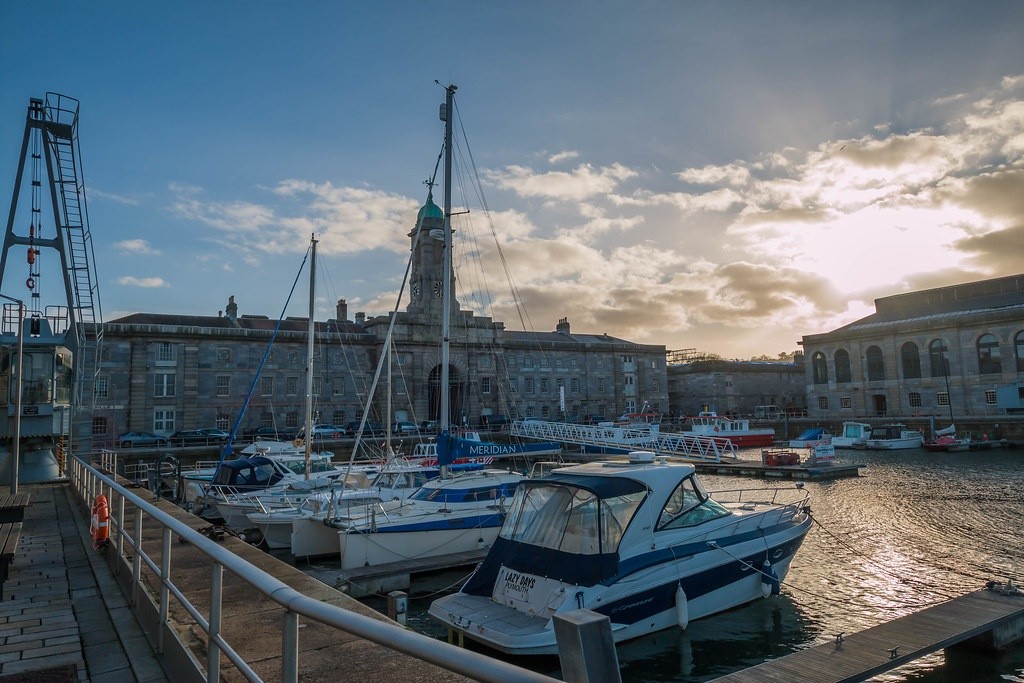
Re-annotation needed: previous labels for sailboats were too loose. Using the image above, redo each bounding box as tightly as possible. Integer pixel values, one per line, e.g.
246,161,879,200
205,84,642,573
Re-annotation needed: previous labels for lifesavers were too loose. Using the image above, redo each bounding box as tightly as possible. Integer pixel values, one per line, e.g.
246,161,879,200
89,494,109,551
292,438,304,449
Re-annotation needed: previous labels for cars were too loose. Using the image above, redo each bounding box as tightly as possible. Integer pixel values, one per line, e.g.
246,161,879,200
311,423,346,437
242,427,295,441
614,413,630,421
117,431,167,447
170,429,223,444
195,427,236,441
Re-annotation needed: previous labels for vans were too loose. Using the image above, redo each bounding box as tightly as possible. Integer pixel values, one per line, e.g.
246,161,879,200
420,419,441,433
585,414,606,425
393,421,421,433
754,406,784,419
346,422,387,438
480,415,511,431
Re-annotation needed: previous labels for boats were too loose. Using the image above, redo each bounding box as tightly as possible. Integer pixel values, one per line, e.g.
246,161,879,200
613,400,776,448
924,340,1007,451
162,231,514,552
427,452,816,658
831,421,872,450
867,423,923,448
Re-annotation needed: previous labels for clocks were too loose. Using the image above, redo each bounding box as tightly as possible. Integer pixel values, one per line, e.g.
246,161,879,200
431,281,444,299
411,282,420,299
